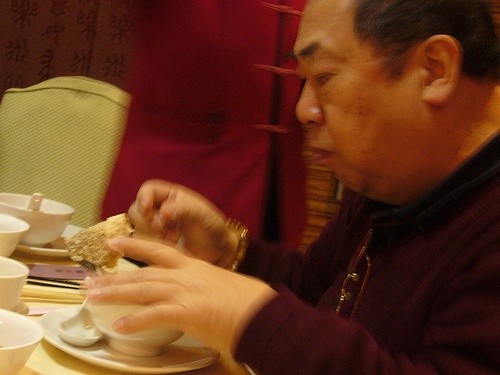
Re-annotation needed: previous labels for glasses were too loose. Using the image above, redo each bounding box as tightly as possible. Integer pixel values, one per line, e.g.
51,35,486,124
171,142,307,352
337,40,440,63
333,230,372,318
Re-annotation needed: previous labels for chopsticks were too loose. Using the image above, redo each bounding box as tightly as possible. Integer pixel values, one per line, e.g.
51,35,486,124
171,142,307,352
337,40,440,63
19,292,85,305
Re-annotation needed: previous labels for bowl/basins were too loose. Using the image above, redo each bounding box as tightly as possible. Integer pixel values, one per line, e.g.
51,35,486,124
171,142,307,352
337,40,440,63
84,299,187,358
0,214,30,258
0,307,45,375
0,256,31,310
0,193,75,247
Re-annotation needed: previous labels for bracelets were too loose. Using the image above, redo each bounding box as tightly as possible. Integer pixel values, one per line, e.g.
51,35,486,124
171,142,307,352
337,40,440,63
225,217,249,273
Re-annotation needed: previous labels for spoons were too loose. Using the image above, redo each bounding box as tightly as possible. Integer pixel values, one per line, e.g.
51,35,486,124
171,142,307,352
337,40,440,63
59,285,106,346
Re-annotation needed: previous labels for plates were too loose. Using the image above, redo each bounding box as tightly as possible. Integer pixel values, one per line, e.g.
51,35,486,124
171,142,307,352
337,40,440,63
40,306,221,373
17,225,90,256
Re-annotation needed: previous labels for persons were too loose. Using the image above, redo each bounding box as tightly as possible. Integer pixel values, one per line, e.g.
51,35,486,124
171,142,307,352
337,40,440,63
84,0,500,375
